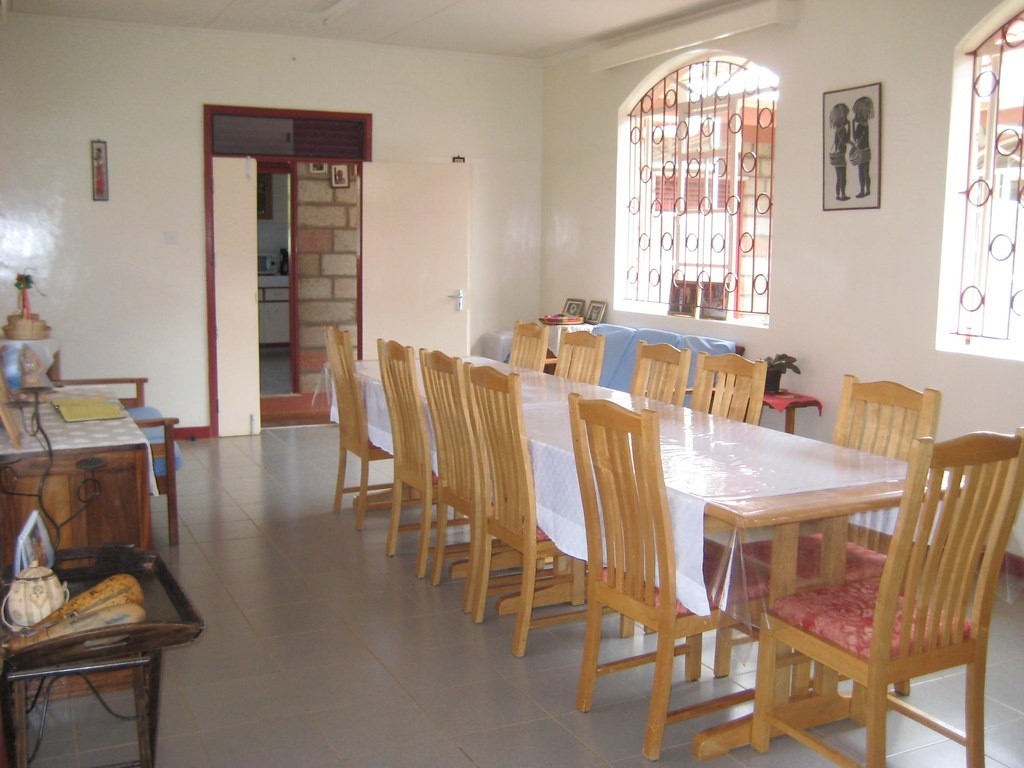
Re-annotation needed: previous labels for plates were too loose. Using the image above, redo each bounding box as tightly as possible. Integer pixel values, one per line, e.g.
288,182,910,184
538,318,584,325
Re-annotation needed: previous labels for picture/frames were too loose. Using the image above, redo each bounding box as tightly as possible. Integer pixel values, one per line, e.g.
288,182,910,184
330,162,352,189
668,279,730,321
583,300,607,324
822,82,881,212
562,298,586,316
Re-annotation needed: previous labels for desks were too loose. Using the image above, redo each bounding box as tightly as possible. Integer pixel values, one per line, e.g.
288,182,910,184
5,646,162,768
330,356,967,761
678,386,823,435
541,324,596,376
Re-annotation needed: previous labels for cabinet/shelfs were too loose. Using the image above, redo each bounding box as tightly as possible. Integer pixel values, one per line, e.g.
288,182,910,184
259,275,290,348
0,385,159,705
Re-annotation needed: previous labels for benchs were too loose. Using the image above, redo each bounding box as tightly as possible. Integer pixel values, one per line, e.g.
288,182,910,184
583,324,745,408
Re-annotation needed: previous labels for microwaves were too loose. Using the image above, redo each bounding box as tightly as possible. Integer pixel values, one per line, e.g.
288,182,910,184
258,253,277,274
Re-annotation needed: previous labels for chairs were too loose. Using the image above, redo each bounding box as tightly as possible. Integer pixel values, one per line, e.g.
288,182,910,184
325,319,1024,768
44,351,184,547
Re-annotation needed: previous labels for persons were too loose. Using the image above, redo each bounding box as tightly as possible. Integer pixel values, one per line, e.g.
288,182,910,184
570,304,576,313
336,170,346,184
674,286,693,312
589,306,597,320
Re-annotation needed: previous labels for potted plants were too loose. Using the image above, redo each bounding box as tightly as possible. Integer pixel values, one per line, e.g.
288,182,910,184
754,353,801,393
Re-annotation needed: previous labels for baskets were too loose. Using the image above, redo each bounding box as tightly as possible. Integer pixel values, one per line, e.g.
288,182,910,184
4,275,52,340
